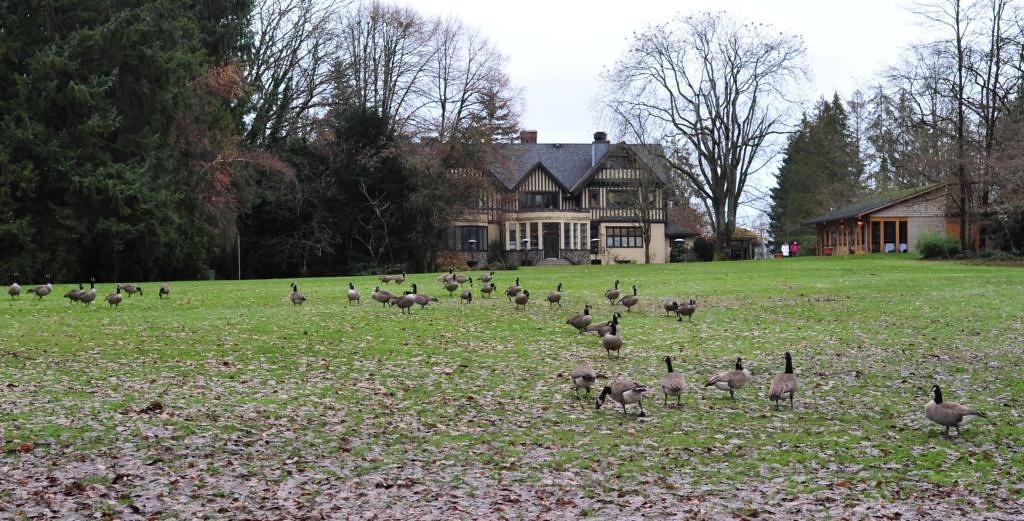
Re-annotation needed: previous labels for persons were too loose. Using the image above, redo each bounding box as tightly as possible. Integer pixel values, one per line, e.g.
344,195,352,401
781,241,790,258
791,241,799,256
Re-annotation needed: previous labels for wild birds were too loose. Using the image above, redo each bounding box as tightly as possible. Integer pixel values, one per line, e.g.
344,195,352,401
6,276,171,310
372,263,798,417
347,281,360,306
289,283,306,306
925,384,986,435
